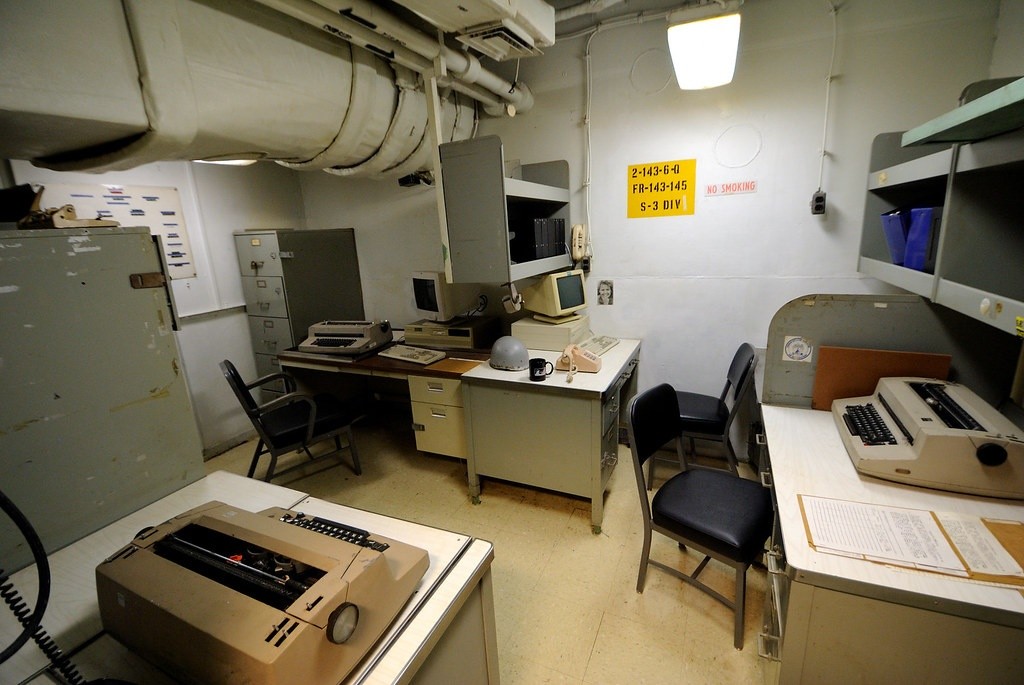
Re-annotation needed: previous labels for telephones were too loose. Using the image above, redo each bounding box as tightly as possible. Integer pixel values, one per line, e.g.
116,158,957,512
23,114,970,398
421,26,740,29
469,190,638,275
555,345,602,373
571,223,585,260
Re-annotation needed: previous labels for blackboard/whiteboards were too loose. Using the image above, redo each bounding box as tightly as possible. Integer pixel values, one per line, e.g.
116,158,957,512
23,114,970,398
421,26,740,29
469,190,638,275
41,181,197,280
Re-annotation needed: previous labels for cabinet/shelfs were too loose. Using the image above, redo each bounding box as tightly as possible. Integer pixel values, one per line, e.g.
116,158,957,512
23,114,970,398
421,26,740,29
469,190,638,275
437,135,574,288
857,115,1024,337
0,226,208,579
231,223,361,394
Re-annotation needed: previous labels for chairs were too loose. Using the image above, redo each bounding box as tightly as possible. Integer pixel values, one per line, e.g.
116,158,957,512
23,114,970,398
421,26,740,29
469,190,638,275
220,360,368,483
627,381,778,648
647,343,758,490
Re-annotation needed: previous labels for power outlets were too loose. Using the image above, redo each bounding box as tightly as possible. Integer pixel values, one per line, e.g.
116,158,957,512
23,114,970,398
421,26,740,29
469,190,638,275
812,191,826,215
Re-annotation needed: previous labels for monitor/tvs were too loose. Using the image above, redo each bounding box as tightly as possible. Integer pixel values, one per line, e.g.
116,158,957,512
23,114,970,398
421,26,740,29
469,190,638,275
411,271,485,327
522,269,588,325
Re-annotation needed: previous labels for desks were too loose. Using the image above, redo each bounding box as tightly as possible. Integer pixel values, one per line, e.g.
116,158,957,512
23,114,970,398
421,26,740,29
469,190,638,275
285,344,491,458
464,337,639,537
760,393,1024,685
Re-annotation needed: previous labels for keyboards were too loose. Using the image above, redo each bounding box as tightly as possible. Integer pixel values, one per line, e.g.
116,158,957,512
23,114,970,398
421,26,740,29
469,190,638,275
378,344,446,364
577,334,619,357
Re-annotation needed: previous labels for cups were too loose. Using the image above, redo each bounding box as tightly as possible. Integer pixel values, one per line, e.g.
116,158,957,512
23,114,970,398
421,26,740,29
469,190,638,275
529,358,554,382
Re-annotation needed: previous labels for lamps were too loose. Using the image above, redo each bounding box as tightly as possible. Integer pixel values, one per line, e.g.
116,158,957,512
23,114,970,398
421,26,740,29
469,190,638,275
665,3,743,91
